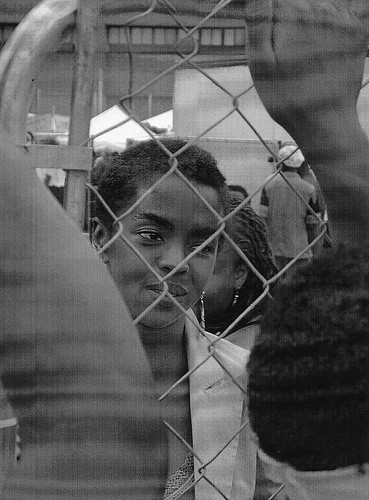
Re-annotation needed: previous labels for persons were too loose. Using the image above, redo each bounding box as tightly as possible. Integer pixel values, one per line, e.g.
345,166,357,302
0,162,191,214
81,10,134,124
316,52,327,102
0,0,369,500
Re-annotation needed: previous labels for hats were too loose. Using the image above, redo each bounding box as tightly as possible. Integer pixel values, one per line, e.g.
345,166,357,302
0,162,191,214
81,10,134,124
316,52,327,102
278,145,305,169
